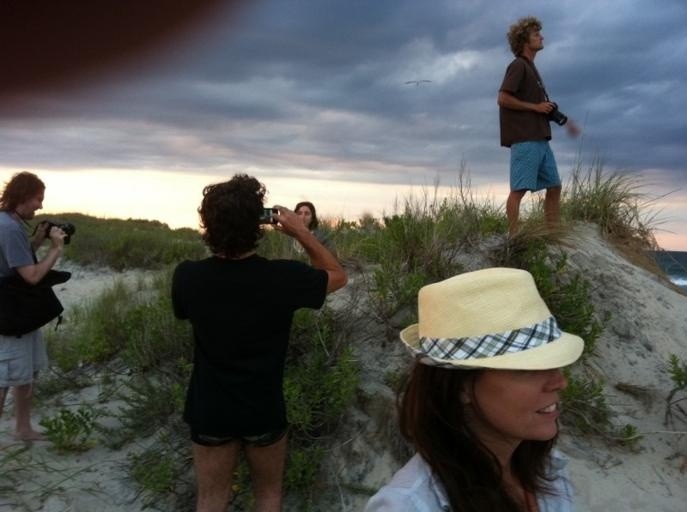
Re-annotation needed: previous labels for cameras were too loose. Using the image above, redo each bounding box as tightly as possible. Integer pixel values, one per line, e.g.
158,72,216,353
545,101,568,126
44,223,77,245
259,207,279,224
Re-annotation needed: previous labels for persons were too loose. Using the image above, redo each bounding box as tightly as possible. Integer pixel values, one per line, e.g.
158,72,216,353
170,175,347,511
362,268,585,512
495,15,563,240
291,203,337,269
0,171,68,442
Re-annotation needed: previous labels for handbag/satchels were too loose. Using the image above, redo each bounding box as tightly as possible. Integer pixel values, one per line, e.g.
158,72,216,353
0,269,71,338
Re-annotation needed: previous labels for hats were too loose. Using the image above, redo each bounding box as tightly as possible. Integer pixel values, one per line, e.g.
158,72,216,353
399,267,584,371
